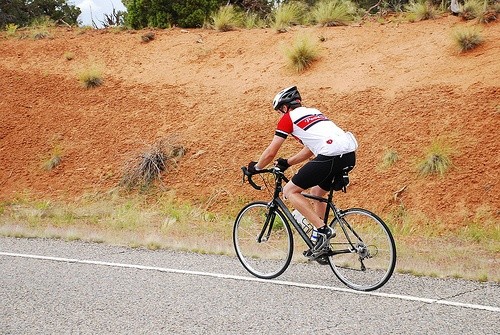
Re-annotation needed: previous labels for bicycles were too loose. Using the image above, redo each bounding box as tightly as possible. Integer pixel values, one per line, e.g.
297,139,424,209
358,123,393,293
233,162,397,293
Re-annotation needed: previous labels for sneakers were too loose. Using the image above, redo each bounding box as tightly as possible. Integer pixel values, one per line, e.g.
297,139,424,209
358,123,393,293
303,249,328,265
314,225,336,250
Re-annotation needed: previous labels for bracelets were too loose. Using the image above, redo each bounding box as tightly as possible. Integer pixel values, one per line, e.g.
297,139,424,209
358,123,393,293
254,164,263,171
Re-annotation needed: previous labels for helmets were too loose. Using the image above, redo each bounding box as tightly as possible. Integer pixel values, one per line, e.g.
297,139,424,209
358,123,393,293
272,86,301,111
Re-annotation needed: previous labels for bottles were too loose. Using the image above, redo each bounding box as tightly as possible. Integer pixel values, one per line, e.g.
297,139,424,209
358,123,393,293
292,209,313,233
310,217,324,241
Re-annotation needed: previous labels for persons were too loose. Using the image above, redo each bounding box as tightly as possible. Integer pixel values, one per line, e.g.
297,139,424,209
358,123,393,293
247,85,358,257
